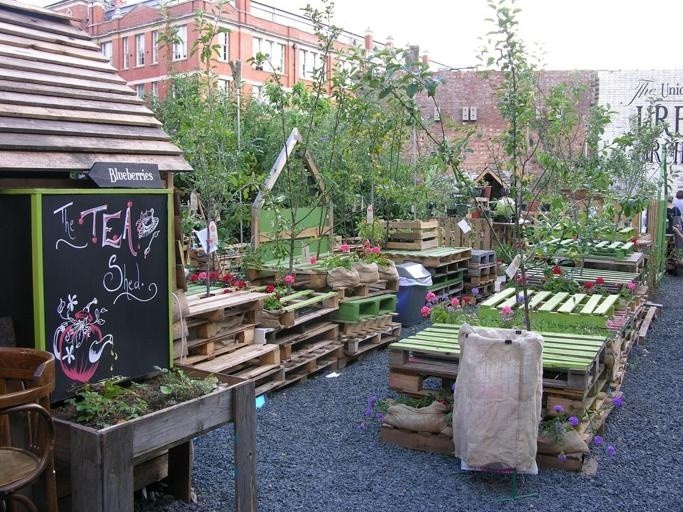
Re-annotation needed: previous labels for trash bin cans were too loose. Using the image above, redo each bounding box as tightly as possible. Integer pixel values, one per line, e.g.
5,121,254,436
392,262,432,328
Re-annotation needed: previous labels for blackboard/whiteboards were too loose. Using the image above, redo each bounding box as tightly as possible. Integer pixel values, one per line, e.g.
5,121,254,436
30,188,175,405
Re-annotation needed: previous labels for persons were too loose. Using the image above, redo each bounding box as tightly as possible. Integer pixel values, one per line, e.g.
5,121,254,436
495,187,517,223
672,189,683,217
666,195,681,254
